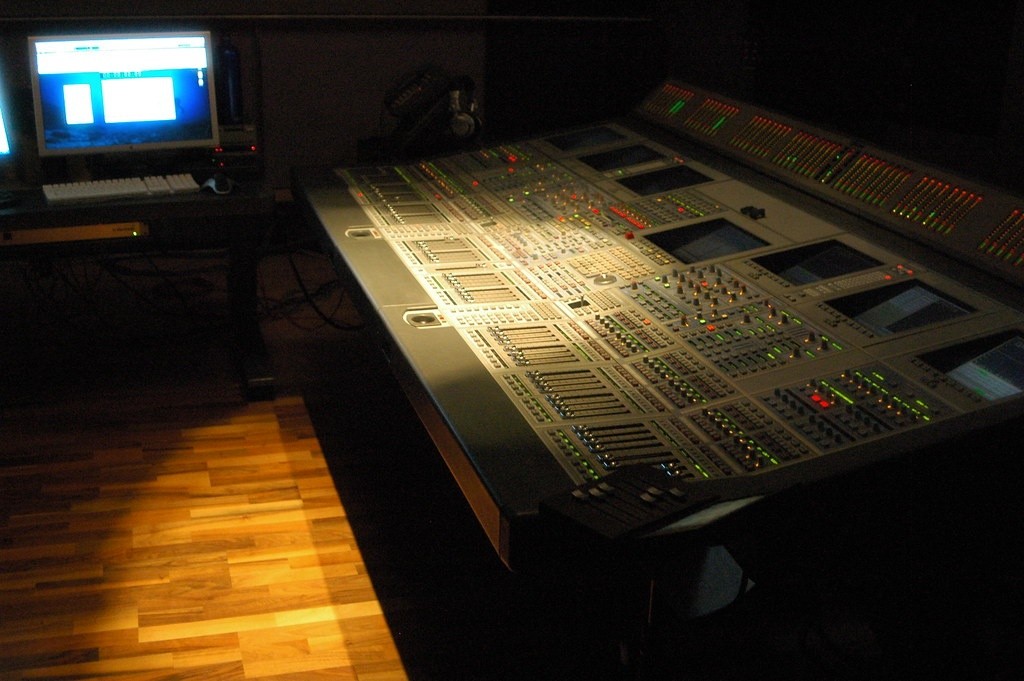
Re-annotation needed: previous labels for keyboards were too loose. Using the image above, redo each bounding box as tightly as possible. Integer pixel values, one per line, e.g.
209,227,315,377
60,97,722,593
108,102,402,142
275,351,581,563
42,173,200,207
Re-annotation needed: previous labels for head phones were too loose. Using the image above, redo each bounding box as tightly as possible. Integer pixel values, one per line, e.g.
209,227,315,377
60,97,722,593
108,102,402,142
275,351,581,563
446,74,485,138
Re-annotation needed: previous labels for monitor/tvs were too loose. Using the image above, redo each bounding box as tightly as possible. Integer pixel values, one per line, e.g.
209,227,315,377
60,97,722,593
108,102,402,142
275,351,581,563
28,29,220,157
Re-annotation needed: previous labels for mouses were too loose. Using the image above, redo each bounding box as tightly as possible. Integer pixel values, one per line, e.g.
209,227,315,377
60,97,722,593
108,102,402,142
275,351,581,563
201,176,233,194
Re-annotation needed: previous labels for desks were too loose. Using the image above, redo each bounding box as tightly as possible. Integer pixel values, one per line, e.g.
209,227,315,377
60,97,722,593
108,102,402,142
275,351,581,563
0,183,278,403
